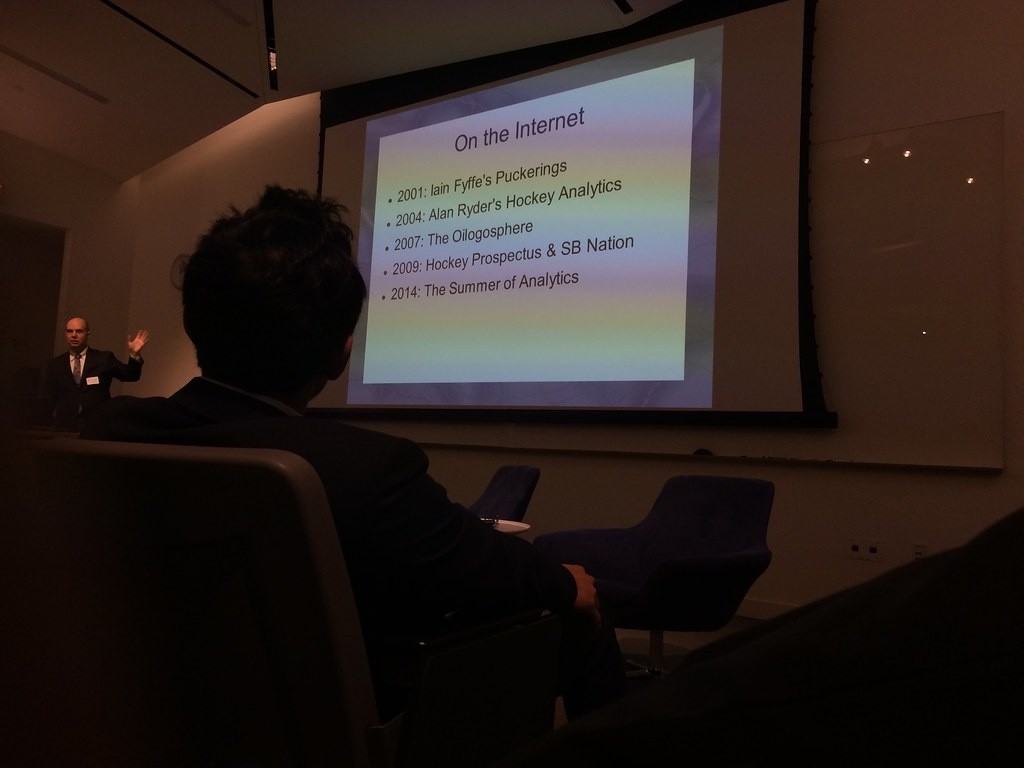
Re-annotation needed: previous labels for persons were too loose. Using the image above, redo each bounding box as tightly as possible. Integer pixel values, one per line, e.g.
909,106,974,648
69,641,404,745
39,317,152,400
78,186,622,725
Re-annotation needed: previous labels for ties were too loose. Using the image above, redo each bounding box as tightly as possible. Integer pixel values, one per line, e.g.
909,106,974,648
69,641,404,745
73,354,81,388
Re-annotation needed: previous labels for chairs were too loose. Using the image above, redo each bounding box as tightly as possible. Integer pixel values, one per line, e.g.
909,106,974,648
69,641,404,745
533,476,775,676
470,464,543,519
1,433,405,767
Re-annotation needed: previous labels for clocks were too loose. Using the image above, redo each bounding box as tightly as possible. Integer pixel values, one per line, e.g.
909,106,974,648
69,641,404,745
171,252,190,290
